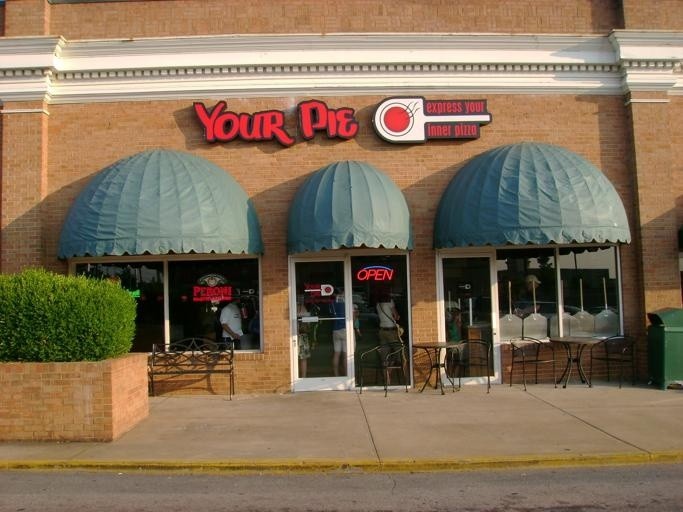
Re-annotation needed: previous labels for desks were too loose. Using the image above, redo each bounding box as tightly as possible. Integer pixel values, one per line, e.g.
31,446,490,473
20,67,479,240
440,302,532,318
412,341,464,396
549,336,601,388
462,321,493,377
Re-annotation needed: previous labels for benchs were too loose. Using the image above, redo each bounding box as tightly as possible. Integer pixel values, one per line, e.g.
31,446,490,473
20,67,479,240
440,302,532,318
146,338,235,402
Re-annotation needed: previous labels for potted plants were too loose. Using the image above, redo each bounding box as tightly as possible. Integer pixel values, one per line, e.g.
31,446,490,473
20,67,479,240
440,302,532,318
0,267,150,442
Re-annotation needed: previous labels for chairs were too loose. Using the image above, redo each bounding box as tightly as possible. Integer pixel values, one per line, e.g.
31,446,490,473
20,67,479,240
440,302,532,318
588,334,638,390
509,336,559,390
360,342,408,397
451,338,492,394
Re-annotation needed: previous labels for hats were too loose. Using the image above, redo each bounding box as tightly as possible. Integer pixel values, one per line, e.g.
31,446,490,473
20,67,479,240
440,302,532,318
524,274,541,284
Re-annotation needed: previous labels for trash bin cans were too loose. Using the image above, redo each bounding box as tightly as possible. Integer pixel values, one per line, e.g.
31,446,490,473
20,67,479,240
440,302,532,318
647,308,683,391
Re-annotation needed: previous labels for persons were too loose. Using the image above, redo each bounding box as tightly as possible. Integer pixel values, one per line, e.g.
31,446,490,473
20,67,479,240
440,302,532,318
446,306,468,378
218,291,245,352
352,302,362,338
328,288,346,377
295,293,312,378
512,274,541,318
375,285,410,386
